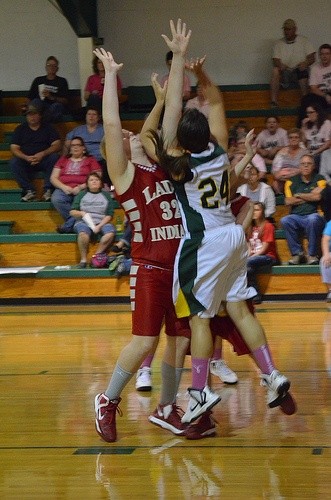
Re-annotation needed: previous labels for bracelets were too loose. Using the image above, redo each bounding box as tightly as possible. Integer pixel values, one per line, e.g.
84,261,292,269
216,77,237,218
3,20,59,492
320,93,326,97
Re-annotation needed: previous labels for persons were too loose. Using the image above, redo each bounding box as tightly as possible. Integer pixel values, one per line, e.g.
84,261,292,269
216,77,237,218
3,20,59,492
160,50,192,101
269,17,316,107
48,136,102,232
236,167,276,224
245,201,280,303
139,52,291,424
64,109,114,190
185,79,211,118
93,18,193,440
232,133,268,182
28,56,71,123
9,106,62,201
114,221,133,253
233,105,331,302
228,120,256,159
135,128,298,439
70,172,115,267
82,54,125,112
297,44,331,128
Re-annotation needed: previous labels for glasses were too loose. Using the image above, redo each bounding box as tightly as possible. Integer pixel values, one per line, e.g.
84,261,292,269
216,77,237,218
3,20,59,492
46,64,57,67
306,111,315,114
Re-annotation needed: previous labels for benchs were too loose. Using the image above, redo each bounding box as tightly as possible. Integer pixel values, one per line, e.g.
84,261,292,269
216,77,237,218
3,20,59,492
0,80,329,299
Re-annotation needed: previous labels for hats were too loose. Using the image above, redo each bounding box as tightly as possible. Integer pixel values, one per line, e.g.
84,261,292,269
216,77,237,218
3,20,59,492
280,18,297,30
24,105,41,114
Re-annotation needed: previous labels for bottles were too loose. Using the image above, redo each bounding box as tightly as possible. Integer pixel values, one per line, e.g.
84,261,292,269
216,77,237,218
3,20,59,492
115,216,122,233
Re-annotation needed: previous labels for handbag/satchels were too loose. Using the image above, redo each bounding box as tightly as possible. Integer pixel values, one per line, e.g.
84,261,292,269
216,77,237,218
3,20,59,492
91,254,107,267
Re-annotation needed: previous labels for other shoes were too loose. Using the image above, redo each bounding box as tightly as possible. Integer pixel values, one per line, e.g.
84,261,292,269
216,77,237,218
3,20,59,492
327,293,331,301
270,101,277,108
252,293,263,304
78,262,86,269
108,255,125,271
307,256,318,265
288,255,303,264
58,227,72,234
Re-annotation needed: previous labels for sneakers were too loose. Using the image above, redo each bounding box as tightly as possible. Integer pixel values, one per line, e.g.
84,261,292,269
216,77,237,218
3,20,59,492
136,366,152,391
21,191,38,201
259,369,290,408
209,360,239,384
185,412,216,439
149,402,185,435
94,392,122,442
42,191,51,201
280,394,296,415
180,385,222,424
